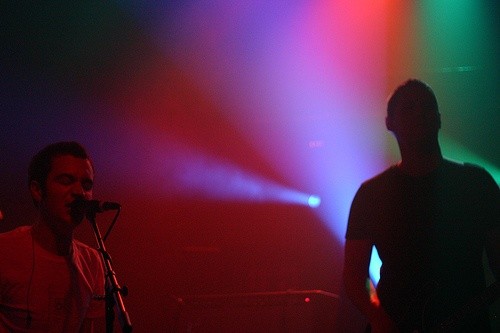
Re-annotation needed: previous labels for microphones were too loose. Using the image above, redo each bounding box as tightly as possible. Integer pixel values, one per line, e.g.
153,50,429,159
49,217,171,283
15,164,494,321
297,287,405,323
71,199,121,212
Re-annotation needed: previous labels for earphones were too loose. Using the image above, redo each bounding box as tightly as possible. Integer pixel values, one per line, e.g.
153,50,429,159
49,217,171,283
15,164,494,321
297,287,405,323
24,315,32,320
